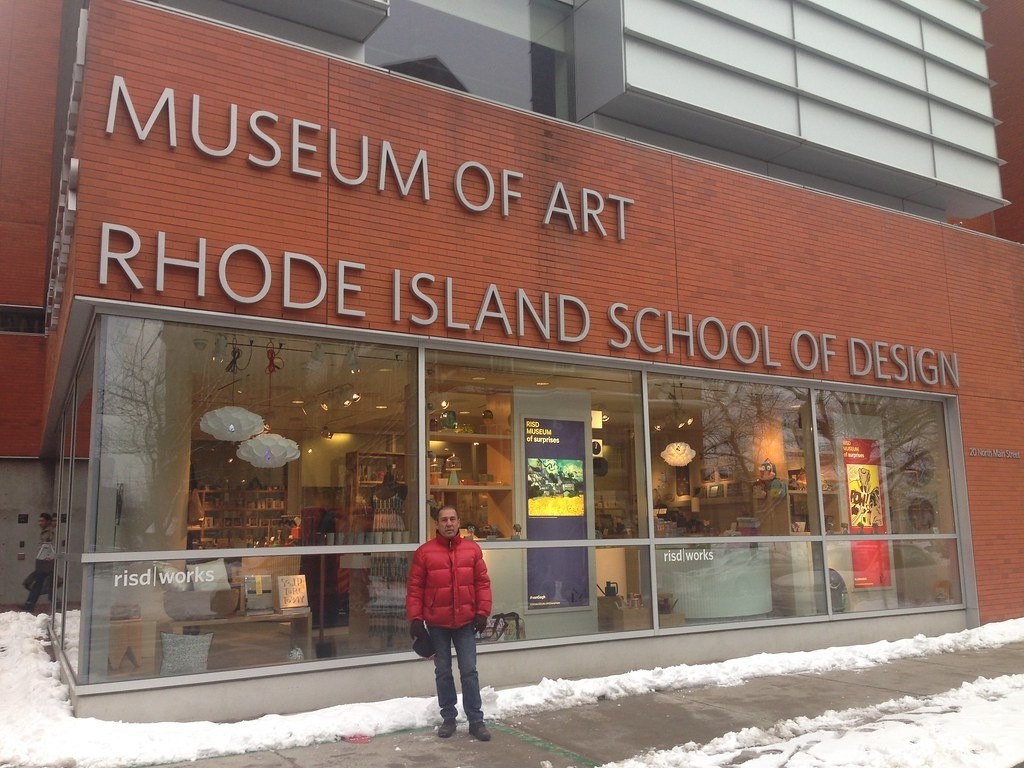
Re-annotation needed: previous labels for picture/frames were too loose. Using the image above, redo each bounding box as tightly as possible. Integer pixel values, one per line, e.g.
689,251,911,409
233,516,242,527
266,497,274,509
692,485,707,499
204,517,213,527
676,464,690,498
224,517,233,528
249,515,259,527
717,464,733,482
700,467,715,483
727,483,739,497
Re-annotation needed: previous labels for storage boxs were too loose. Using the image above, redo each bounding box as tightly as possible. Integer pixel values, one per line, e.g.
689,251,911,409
276,575,309,609
107,618,144,676
598,595,624,621
613,606,651,635
657,612,686,629
479,474,493,482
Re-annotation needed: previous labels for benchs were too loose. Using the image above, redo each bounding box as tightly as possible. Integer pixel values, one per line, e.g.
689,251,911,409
158,610,312,675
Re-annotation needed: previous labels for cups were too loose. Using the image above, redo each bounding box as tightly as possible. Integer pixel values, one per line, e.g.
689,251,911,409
393,531,402,543
438,478,448,485
374,531,383,543
402,531,410,543
335,533,344,544
383,532,392,543
366,532,374,544
326,533,334,545
796,522,806,532
356,532,364,544
345,532,354,544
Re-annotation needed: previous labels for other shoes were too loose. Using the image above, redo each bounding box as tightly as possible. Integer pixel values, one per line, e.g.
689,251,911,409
19,606,33,611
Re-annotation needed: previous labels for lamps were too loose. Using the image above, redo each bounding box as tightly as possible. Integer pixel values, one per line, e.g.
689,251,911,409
660,383,697,468
196,334,265,443
237,337,301,470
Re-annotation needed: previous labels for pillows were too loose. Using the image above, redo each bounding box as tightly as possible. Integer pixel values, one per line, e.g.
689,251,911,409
160,631,213,676
152,560,193,592
187,557,231,591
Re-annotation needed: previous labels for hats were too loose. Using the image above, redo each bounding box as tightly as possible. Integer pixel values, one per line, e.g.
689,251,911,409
410,628,435,657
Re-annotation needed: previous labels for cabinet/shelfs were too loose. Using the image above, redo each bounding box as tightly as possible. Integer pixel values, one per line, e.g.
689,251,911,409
188,489,287,544
405,377,513,540
765,408,899,615
345,451,458,537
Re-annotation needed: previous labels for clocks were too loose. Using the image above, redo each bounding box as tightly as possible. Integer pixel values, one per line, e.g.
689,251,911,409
592,438,603,458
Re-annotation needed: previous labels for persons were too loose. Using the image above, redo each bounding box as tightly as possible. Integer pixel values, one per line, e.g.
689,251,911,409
405,505,492,741
19,512,58,611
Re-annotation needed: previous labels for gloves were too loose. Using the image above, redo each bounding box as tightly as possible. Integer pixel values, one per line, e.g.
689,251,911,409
472,614,488,634
410,619,424,641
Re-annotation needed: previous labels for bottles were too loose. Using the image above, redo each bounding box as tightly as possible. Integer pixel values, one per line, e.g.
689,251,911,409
691,496,700,512
656,518,665,538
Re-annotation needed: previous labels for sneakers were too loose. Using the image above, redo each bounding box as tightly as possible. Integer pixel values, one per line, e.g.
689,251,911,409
438,718,457,737
469,721,491,742
